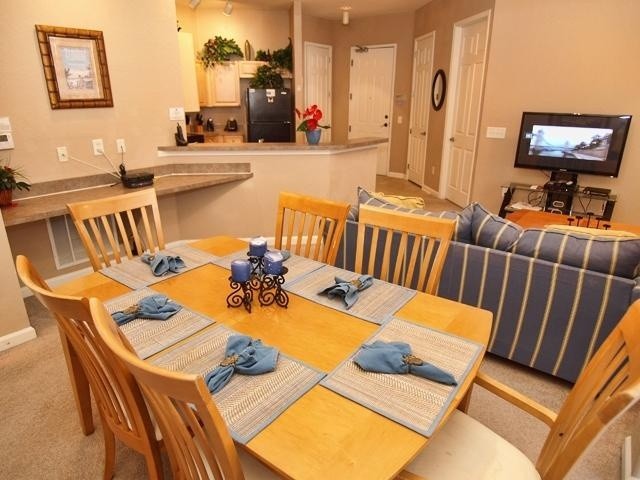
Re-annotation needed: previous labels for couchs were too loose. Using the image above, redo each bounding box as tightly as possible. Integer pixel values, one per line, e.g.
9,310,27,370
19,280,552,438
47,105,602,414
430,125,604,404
321,205,640,383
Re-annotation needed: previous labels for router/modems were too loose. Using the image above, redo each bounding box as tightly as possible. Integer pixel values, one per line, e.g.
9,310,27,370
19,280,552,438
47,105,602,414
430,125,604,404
583,186,612,198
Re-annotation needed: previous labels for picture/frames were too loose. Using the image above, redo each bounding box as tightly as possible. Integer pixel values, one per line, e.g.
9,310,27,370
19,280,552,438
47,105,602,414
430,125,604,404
35,24,115,110
432,69,447,111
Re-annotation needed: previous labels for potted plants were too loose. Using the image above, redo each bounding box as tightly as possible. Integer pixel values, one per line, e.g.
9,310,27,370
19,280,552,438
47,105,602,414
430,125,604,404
0,156,31,207
198,36,243,69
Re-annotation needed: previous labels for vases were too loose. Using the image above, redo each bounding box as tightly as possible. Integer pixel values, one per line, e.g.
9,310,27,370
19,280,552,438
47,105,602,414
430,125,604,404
306,129,322,144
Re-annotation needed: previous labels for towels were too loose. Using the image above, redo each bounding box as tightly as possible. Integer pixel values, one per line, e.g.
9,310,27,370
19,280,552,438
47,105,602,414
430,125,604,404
317,275,373,311
204,335,279,395
353,340,458,387
281,249,290,260
111,295,183,326
141,252,186,277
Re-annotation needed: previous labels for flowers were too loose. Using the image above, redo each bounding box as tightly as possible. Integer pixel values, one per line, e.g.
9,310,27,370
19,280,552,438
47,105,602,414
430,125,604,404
296,105,331,132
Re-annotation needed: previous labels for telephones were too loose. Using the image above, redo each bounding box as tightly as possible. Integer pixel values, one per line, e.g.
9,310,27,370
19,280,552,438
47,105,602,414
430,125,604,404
175,123,188,146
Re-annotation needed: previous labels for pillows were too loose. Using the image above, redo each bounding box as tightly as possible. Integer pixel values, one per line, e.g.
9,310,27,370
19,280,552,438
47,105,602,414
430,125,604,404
546,225,638,241
473,203,640,278
370,190,425,210
358,187,474,243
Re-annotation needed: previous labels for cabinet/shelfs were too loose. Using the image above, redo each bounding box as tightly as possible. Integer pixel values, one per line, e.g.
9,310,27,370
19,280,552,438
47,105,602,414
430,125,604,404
241,61,265,78
197,60,239,107
179,33,200,112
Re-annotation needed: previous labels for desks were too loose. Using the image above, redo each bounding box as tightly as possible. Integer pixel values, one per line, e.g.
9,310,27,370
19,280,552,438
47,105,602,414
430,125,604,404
0,163,253,228
499,183,617,220
508,209,640,240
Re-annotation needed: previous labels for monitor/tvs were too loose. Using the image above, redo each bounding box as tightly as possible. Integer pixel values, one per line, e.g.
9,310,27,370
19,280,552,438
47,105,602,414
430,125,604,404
515,111,633,192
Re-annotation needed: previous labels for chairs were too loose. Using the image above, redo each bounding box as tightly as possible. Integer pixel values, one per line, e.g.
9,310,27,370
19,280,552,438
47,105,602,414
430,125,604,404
355,203,457,296
274,191,350,266
16,254,204,480
65,187,166,270
391,300,639,480
91,295,286,478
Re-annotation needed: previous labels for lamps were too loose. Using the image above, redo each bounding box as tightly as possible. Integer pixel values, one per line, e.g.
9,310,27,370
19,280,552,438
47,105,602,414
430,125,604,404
339,6,352,26
190,1,200,9
223,1,233,16
